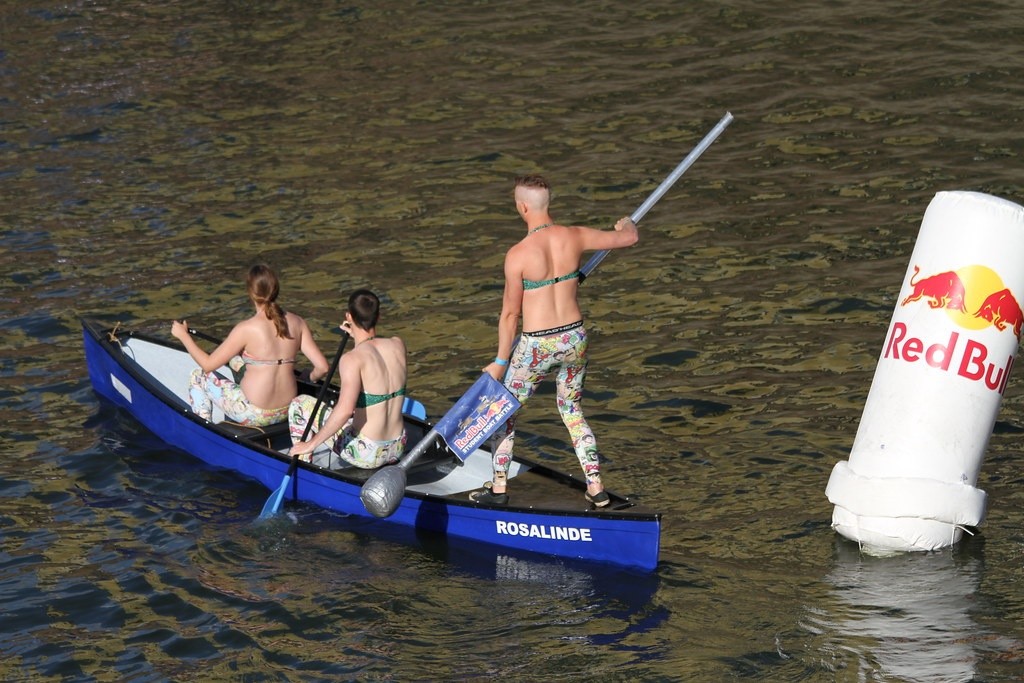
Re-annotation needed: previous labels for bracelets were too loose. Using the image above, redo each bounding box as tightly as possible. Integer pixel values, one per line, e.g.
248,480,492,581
495,357,510,366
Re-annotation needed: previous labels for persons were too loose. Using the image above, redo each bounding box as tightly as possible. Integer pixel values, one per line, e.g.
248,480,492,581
468,174,638,506
171,265,330,428
290,289,407,470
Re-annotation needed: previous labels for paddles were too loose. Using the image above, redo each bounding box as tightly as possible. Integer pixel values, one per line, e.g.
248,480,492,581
255,321,352,525
186,330,302,377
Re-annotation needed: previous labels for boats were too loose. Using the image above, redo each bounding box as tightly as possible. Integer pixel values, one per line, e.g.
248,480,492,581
80,318,661,570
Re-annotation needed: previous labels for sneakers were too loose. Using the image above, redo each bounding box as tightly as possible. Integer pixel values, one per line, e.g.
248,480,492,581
469,481,509,506
585,490,610,508
